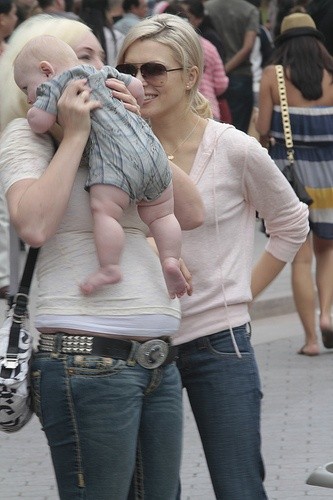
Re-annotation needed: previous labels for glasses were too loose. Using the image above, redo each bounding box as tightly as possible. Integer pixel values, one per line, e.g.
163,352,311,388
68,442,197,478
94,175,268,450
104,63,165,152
115,62,185,87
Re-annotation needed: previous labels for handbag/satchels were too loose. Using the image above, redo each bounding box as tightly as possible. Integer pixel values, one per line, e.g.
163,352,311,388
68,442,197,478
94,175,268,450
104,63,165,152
0,321,32,433
260,164,313,238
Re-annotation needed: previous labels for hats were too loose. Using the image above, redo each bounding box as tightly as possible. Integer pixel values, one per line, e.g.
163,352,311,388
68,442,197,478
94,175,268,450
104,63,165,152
274,11,322,47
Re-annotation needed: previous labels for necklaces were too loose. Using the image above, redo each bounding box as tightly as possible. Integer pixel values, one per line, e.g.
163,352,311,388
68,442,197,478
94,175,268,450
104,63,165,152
164,117,201,161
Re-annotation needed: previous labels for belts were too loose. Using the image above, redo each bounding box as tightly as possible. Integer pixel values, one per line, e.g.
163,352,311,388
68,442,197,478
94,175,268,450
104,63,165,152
32,333,174,369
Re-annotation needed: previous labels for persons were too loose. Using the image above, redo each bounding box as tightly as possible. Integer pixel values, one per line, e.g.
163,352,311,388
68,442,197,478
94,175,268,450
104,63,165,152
0,1,333,357
115,13,310,500
0,13,206,500
